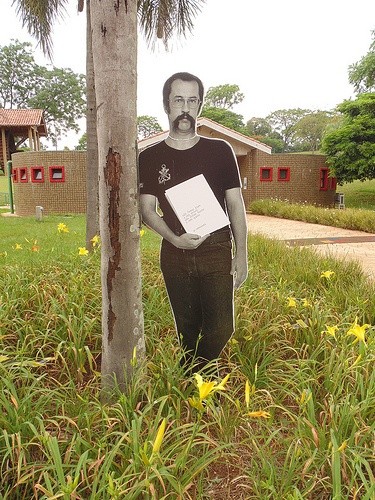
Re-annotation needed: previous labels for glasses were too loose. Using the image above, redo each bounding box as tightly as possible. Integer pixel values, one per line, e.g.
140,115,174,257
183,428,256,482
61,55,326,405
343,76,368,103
169,97,201,109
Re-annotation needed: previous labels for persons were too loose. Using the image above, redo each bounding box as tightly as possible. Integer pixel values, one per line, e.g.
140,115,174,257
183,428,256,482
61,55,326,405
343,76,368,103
139,72,248,407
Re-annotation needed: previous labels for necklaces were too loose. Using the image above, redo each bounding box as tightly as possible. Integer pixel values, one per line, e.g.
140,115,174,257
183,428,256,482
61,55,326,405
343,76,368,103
168,134,197,141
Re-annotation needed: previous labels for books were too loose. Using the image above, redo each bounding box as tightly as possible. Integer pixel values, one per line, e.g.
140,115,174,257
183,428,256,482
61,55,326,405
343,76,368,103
164,174,231,239
